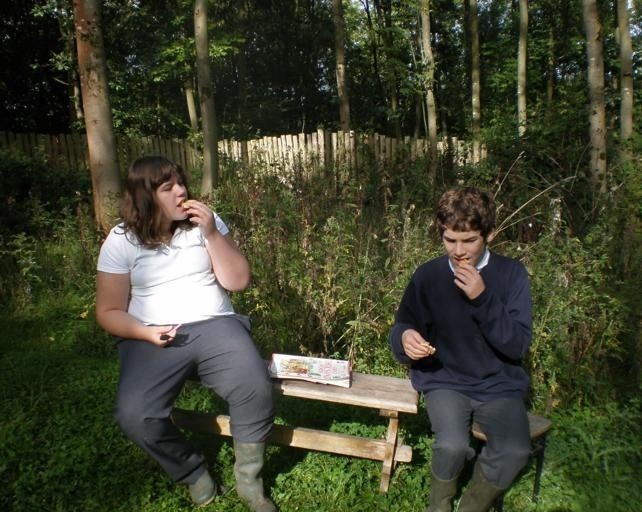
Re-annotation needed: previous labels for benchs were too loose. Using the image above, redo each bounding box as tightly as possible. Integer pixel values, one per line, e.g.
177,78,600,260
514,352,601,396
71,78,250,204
171,358,420,497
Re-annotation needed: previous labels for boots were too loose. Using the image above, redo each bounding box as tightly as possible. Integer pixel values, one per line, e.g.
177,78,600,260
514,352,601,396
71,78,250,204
234,439,278,512
426,466,459,512
188,468,218,506
456,462,507,512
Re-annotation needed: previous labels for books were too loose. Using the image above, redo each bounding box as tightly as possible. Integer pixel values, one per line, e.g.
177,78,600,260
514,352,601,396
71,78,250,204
268,353,349,388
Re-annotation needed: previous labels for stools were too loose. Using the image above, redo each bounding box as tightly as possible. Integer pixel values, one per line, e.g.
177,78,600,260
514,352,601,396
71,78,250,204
471,408,554,511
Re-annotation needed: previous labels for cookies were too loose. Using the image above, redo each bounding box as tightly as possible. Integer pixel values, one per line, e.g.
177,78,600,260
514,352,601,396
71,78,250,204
180,200,188,206
459,259,470,264
421,343,436,355
168,328,176,338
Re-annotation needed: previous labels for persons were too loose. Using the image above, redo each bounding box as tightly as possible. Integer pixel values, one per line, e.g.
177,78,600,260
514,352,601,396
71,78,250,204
391,187,532,511
96,156,275,512
